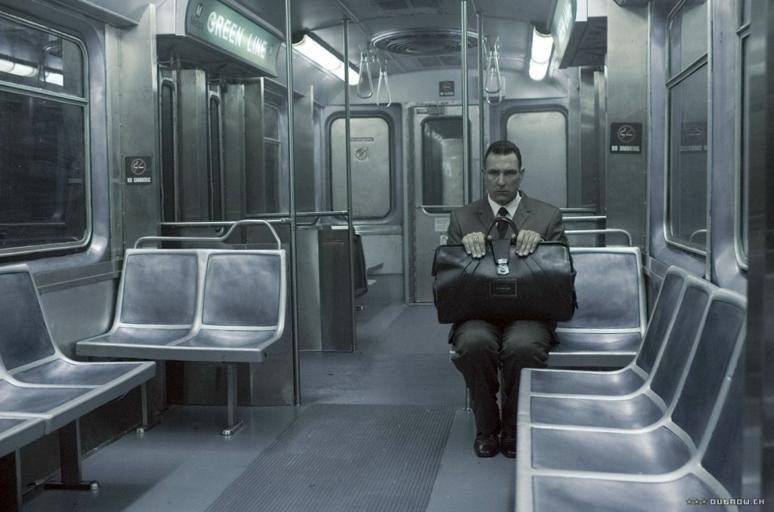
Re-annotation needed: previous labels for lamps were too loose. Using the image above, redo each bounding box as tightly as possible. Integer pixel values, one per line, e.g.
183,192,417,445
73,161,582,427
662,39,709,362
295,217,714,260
525,24,554,83
289,28,365,89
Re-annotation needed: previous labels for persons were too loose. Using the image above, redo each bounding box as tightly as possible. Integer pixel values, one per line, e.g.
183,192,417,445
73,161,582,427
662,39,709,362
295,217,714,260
441,138,575,458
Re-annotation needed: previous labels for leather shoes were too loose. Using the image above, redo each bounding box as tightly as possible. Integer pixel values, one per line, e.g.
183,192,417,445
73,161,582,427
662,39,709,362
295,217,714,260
501,431,516,458
474,431,499,457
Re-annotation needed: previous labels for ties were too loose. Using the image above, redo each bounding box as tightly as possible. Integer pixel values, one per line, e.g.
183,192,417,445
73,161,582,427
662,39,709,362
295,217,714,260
497,208,508,238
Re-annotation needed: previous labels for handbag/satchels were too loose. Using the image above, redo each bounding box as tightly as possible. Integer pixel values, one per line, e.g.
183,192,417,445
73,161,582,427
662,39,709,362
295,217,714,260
432,217,579,323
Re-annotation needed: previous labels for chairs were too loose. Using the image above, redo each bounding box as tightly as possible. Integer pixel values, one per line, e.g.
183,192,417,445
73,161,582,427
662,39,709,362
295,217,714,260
0,238,286,491
512,244,750,512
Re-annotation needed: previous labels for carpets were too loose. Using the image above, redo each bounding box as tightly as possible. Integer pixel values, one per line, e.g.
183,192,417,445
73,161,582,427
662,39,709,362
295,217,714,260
203,402,458,512
